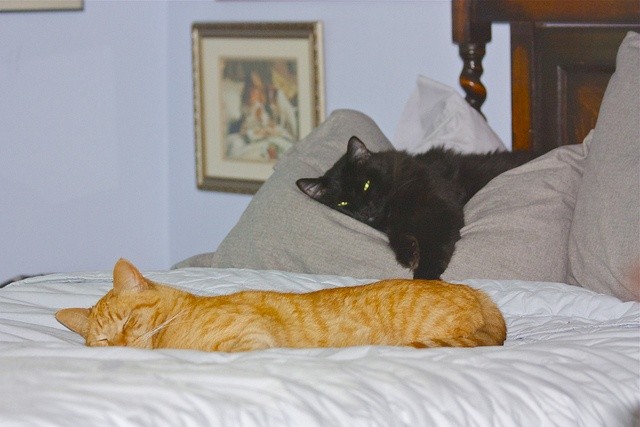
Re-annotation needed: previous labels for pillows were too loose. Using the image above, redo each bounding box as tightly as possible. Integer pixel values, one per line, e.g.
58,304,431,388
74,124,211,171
208,109,595,284
563,29,639,303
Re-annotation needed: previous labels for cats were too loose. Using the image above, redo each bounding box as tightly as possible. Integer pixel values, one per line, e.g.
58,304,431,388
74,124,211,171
55,257,508,354
296,135,548,280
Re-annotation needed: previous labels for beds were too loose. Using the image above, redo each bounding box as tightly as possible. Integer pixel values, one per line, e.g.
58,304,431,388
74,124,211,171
1,251,638,427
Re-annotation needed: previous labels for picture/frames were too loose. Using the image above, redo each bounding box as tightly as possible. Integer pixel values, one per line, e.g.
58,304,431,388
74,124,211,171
191,21,325,195
